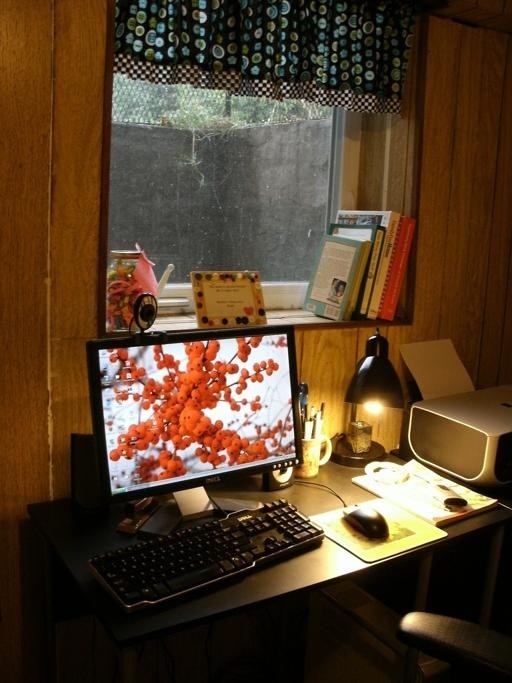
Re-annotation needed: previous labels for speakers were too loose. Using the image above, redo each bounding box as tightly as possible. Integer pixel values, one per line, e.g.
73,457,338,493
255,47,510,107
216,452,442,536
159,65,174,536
70,432,112,520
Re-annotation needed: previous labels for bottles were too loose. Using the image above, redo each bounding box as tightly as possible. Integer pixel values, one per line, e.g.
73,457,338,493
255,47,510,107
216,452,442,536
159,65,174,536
106,249,155,332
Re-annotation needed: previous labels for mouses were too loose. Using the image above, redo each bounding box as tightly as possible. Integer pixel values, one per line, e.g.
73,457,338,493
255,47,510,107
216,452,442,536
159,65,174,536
342,504,390,538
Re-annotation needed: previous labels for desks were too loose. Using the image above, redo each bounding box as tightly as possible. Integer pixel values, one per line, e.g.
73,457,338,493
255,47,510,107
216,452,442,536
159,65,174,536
27,454,512,683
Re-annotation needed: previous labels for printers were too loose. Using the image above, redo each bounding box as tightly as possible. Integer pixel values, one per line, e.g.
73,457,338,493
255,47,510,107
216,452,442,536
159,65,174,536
408,383,512,490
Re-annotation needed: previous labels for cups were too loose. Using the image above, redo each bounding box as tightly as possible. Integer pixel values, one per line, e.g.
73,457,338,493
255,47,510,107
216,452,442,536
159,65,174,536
294,439,333,479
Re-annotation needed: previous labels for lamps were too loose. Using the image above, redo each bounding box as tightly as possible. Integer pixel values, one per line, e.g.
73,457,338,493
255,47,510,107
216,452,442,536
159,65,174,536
328,328,404,468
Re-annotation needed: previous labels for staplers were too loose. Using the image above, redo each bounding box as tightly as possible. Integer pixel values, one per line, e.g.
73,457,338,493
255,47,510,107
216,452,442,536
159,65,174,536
115,496,162,535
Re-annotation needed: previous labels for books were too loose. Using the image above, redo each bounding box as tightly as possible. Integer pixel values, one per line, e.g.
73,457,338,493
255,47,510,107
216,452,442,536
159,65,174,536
351,459,499,527
304,209,417,322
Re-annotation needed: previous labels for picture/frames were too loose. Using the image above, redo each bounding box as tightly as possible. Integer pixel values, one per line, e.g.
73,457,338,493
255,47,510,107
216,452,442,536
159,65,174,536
190,271,269,328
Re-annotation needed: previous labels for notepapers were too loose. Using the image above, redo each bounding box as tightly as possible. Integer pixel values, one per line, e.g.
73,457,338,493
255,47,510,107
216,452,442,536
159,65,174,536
172,486,215,521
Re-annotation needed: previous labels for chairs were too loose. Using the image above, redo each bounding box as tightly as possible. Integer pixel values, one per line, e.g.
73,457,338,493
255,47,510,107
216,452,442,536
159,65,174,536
397,612,512,683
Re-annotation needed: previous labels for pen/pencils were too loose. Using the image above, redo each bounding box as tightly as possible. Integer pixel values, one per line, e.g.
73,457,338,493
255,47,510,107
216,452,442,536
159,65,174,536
299,382,324,440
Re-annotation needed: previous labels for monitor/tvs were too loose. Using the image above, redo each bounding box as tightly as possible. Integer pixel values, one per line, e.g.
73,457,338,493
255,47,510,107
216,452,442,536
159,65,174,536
86,325,303,537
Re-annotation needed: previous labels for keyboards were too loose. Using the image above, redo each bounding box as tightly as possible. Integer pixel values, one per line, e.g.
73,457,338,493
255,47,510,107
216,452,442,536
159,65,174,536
87,498,324,615
